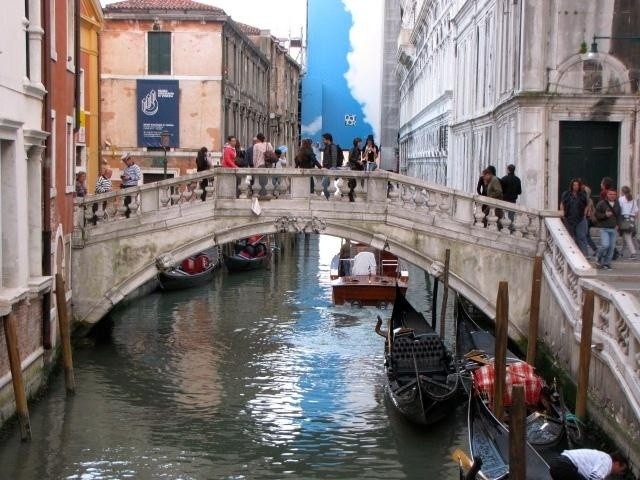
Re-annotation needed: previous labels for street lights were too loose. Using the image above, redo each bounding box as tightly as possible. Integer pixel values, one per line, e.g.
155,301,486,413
587,34,639,61
159,124,171,180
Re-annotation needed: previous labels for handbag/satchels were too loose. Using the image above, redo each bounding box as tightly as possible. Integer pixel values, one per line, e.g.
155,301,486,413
619,219,631,232
263,142,279,168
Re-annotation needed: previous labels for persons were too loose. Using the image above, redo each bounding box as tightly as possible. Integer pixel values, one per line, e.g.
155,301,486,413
92,169,113,225
220,133,379,203
477,164,522,234
550,448,629,480
560,177,639,270
120,153,142,218
75,173,87,226
195,147,213,202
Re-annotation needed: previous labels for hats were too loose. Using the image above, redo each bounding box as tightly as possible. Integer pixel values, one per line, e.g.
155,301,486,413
121,153,130,161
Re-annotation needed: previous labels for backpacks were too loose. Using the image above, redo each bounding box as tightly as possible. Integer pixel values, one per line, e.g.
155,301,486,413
329,143,344,167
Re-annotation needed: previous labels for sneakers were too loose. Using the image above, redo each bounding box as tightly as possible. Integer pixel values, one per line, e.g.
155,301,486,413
600,265,613,271
595,253,602,266
626,255,637,260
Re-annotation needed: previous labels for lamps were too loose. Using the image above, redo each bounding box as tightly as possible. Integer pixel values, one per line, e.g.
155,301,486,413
589,34,639,55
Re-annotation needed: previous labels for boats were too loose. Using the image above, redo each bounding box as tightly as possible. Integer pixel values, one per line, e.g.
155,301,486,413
375,276,459,429
222,241,275,275
453,289,573,448
156,246,220,292
464,379,555,480
327,236,411,310
